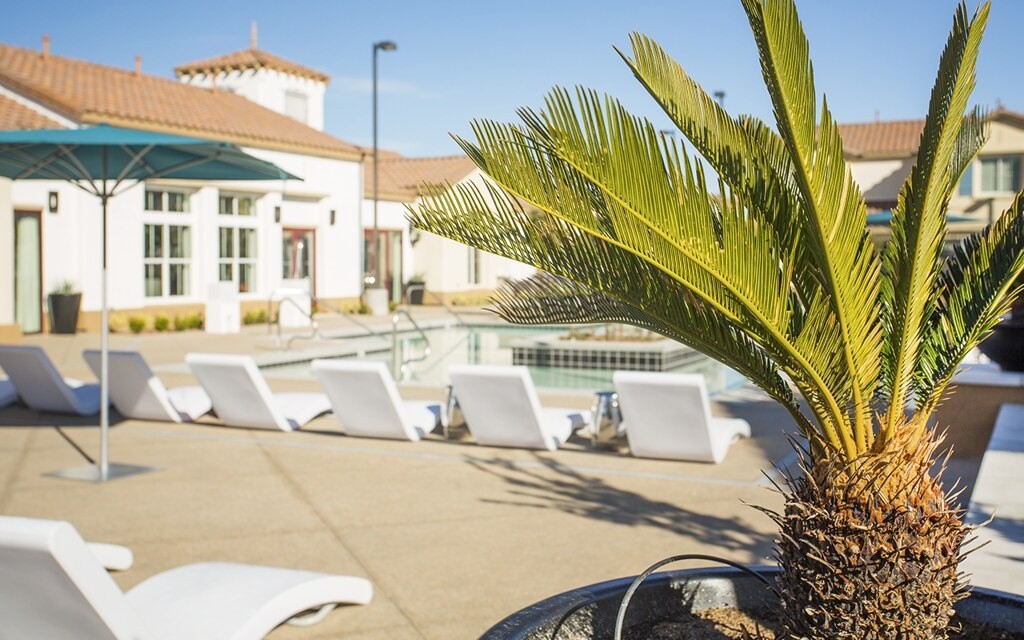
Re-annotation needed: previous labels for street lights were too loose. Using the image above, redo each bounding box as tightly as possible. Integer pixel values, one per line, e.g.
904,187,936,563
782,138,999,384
713,92,726,194
370,40,398,292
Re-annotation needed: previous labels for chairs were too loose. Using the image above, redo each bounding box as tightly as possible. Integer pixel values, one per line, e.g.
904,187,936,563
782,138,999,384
612,369,750,464
0,515,374,640
310,359,442,441
0,381,18,408
0,346,112,416
82,351,211,423
187,353,331,431
86,543,134,571
449,365,592,451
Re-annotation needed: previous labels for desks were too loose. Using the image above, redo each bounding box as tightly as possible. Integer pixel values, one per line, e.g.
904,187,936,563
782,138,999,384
594,392,621,435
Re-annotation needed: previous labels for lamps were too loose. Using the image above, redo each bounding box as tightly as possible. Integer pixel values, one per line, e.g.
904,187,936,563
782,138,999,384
49,192,58,211
331,211,335,224
275,206,280,221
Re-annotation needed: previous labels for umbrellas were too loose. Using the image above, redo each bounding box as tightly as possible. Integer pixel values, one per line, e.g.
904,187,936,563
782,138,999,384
865,208,978,226
0,123,304,481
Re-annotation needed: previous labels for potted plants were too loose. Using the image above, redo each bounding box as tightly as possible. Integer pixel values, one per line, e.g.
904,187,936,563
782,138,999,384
49,283,81,334
403,0,1024,639
407,273,425,305
